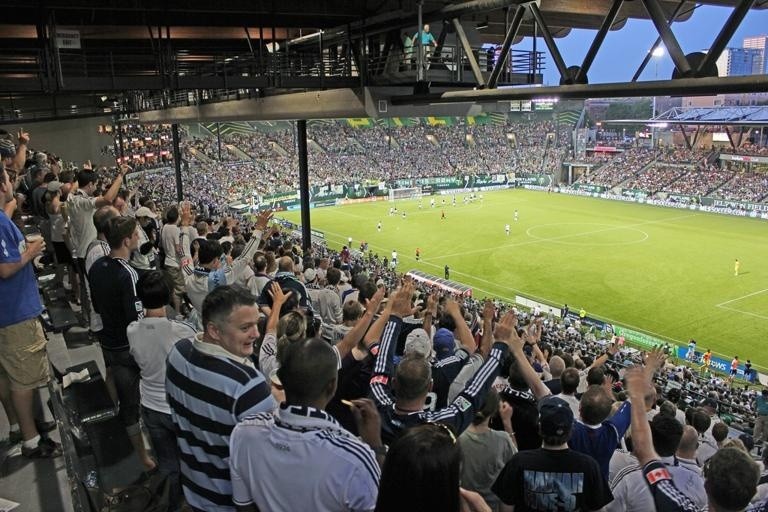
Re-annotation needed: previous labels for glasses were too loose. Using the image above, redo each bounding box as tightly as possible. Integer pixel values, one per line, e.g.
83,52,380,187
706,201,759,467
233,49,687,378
423,419,460,444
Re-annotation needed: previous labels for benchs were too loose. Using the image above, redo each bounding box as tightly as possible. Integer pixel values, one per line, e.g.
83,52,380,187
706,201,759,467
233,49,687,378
12,204,160,510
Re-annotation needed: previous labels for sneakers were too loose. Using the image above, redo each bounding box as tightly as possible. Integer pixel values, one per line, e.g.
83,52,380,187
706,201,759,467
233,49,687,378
145,454,159,478
21,435,62,458
9,418,57,443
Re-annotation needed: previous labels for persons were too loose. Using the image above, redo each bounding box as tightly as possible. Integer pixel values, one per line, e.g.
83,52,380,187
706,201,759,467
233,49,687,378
412,24,437,65
1,127,767,511
402,33,413,71
487,47,495,72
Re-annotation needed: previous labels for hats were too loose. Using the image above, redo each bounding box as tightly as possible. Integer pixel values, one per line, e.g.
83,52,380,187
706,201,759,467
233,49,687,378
134,206,158,220
404,328,431,358
254,275,313,309
433,327,457,356
537,395,574,437
47,180,64,192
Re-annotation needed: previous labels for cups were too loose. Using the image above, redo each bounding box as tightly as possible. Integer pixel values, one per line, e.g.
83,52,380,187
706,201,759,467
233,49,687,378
24,232,42,244
